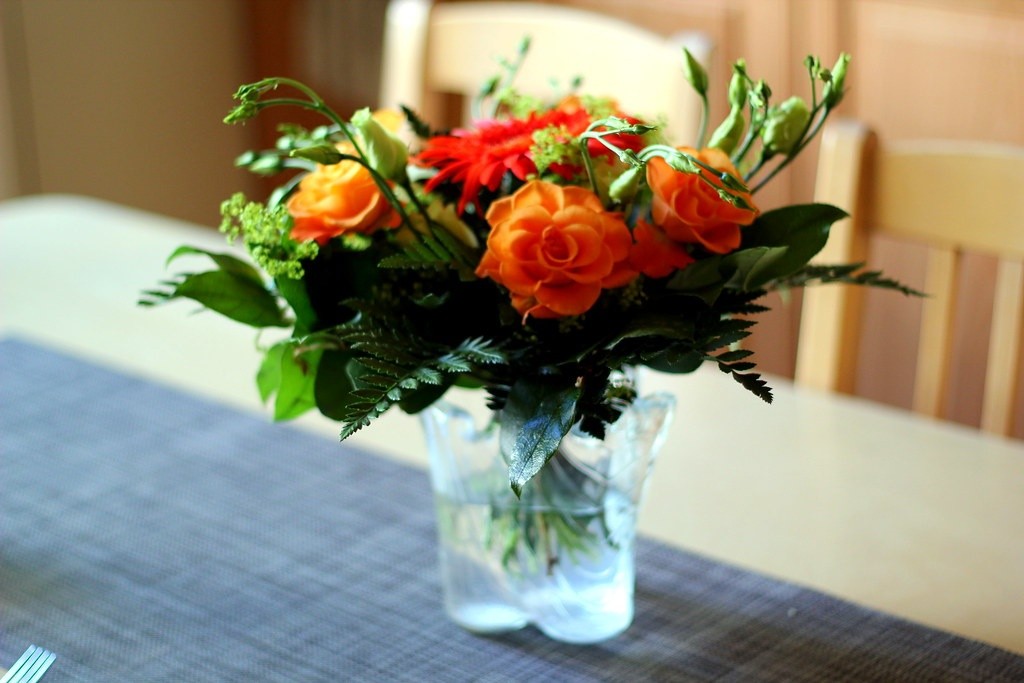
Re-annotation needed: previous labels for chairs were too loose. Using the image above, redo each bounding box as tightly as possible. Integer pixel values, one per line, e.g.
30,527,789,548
793,117,1023,442
379,0,712,153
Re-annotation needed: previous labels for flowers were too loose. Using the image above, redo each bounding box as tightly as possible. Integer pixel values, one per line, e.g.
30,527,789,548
137,40,929,572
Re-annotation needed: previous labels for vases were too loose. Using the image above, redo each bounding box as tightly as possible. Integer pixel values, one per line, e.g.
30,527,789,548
414,360,677,647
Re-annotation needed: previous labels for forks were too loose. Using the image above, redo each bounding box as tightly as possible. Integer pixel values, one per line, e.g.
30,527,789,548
0,645,57,683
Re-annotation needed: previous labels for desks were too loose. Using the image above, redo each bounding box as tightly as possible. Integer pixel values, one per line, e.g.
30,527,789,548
0,193,1022,683
